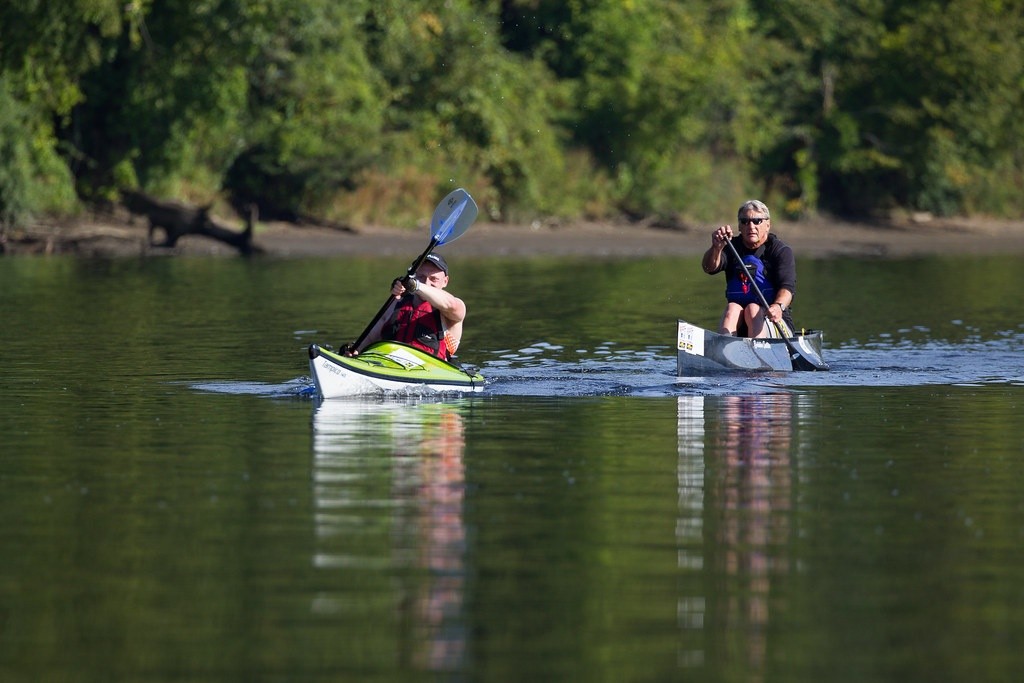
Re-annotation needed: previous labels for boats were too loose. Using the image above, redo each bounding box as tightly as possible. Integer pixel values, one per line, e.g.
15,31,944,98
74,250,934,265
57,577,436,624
674,316,826,375
306,336,486,398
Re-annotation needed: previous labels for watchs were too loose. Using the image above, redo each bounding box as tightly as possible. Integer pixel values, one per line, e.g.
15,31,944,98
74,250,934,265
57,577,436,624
773,302,786,311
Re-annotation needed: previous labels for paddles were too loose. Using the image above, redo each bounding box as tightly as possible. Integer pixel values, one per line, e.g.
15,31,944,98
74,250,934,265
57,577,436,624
722,236,835,373
347,186,481,356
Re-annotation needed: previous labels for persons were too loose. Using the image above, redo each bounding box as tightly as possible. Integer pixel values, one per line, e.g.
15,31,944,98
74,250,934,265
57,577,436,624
702,200,796,338
339,253,467,362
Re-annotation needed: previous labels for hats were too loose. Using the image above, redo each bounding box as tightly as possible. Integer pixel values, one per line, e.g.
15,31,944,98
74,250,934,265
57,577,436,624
412,252,448,276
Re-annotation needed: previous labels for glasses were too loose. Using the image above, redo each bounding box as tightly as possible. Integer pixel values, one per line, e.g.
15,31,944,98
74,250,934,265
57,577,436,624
739,217,768,227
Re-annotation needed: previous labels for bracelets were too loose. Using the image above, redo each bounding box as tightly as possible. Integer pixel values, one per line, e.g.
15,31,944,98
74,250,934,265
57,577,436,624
407,278,420,294
